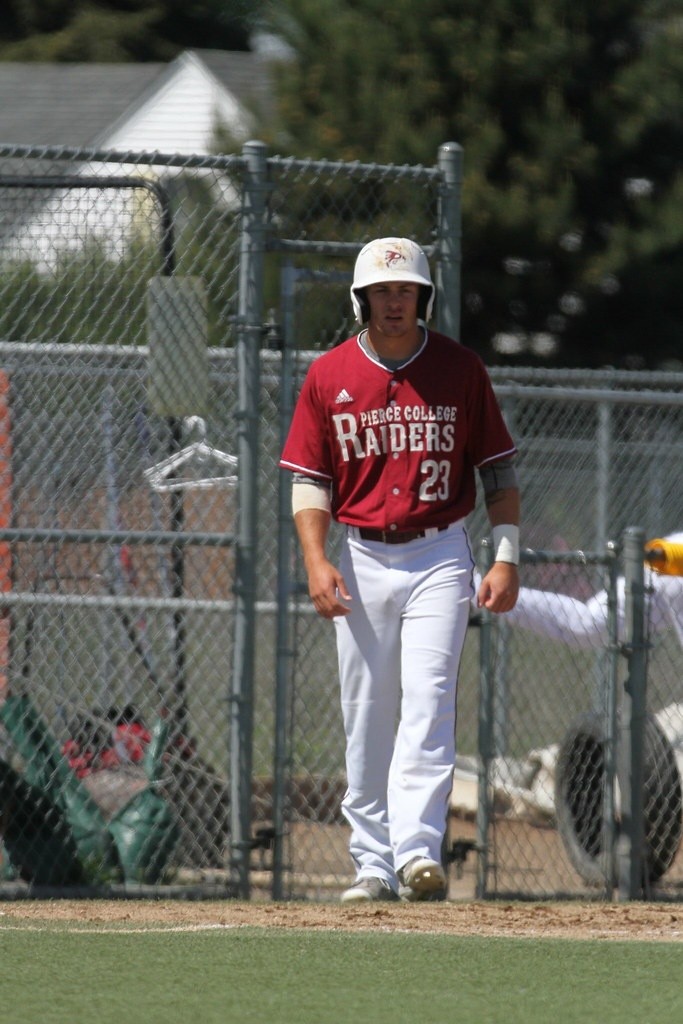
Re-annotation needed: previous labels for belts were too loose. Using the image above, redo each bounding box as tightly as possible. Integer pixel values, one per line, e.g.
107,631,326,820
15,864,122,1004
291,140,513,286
360,523,450,545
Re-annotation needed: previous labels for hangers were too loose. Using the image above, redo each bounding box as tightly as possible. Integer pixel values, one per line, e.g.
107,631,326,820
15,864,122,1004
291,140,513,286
140,415,238,493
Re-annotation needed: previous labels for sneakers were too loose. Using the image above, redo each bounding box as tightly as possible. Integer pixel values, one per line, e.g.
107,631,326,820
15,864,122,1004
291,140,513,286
397,857,447,901
340,876,398,903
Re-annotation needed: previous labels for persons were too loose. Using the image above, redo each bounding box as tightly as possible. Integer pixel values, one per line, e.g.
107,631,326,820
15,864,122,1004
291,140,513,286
277,236,521,901
471,530,683,651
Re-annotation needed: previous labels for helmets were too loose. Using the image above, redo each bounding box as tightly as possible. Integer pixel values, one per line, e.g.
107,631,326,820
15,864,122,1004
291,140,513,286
350,236,436,325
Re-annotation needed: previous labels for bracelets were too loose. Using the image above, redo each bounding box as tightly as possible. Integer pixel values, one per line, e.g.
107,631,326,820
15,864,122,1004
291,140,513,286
493,524,520,566
